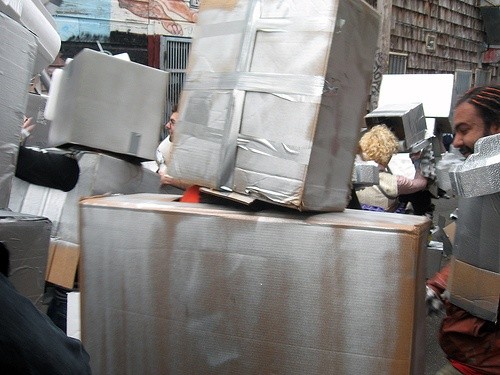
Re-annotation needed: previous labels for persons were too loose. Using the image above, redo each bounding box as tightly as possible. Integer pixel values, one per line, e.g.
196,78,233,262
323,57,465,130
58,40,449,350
353,125,428,215
422,83,500,374
149,105,205,196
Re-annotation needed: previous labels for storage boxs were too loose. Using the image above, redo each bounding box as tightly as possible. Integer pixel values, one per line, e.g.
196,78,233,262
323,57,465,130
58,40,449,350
3,0,500,375
